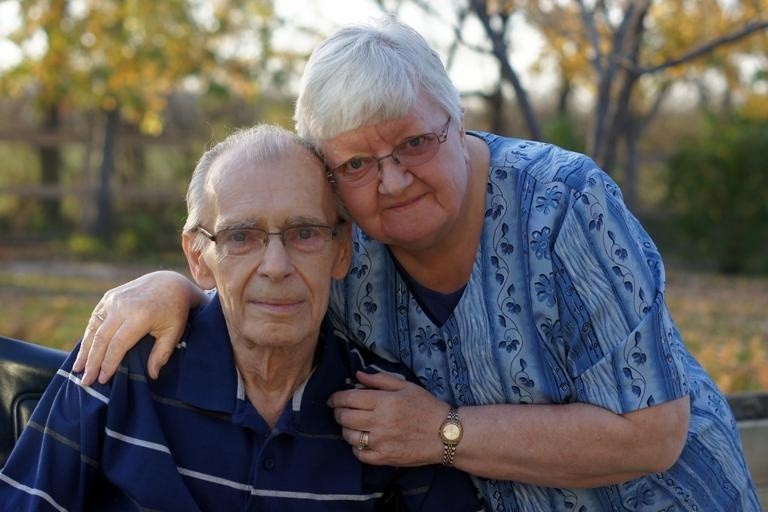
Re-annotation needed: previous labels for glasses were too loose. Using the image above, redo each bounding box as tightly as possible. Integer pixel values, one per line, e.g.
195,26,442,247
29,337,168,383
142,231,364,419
320,113,455,188
191,211,346,256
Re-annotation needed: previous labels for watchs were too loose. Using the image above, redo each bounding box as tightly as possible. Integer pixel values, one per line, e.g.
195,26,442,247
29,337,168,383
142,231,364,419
438,405,463,466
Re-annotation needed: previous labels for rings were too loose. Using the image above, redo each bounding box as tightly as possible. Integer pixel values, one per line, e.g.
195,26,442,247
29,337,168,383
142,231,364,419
359,431,369,448
94,312,104,322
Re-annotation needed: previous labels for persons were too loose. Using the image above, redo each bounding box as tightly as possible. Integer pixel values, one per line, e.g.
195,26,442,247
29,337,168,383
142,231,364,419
0,121,477,512
71,16,760,511
